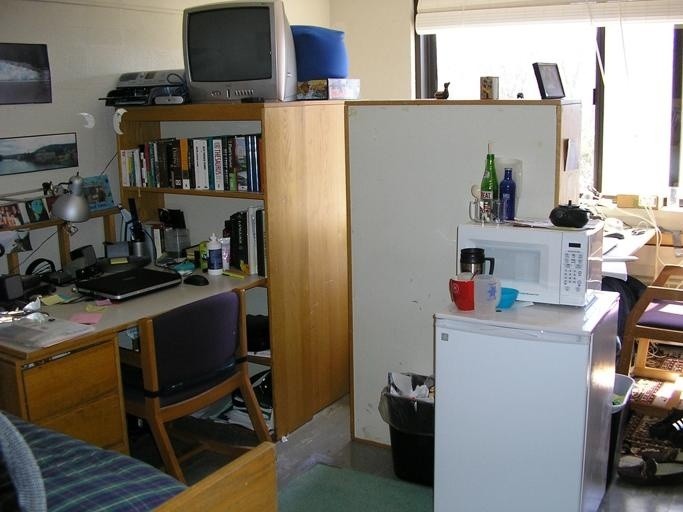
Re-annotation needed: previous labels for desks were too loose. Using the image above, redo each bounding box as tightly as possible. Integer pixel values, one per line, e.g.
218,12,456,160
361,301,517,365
0,256,261,453
552,227,656,359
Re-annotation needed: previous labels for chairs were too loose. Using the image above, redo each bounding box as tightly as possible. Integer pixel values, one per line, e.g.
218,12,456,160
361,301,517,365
119,289,270,488
624,264,683,383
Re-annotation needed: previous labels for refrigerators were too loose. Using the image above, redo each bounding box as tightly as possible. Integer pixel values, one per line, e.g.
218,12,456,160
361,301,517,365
431,291,621,512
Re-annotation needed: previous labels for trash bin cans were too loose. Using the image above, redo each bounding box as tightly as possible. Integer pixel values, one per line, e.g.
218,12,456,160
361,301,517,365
608,374,635,468
378,373,435,487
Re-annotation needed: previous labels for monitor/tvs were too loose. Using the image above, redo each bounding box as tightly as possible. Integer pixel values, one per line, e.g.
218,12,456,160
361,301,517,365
184,2,280,101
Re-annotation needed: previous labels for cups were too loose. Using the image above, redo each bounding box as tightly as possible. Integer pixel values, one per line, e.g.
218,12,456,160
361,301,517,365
473,274,501,316
468,198,480,222
448,272,477,311
459,248,494,275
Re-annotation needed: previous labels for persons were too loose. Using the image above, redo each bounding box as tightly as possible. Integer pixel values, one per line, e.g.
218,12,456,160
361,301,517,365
27,199,48,222
297,83,313,99
0,205,24,227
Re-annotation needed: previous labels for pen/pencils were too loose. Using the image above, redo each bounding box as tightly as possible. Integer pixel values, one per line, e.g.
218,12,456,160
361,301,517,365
222,271,246,279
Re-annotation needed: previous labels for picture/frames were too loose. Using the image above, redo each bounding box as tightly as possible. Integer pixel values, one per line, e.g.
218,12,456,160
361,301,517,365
83,175,115,212
0,132,78,176
533,63,565,98
5,191,54,202
18,198,51,223
0,43,52,105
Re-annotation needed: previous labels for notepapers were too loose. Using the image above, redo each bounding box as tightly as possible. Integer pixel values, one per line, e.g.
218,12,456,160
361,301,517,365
59,293,74,301
96,298,112,305
41,294,64,306
86,303,108,313
69,312,103,324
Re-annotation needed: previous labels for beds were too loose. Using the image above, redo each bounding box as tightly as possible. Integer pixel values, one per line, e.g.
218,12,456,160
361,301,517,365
0,409,276,511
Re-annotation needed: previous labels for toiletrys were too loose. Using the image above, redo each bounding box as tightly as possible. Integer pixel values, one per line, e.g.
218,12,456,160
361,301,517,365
200,241,209,273
222,238,231,271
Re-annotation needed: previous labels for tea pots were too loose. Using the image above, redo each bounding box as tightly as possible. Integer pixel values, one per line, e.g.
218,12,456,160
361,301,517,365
548,201,594,230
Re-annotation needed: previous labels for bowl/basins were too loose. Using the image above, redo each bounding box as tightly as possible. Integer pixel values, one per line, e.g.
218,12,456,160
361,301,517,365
495,287,518,309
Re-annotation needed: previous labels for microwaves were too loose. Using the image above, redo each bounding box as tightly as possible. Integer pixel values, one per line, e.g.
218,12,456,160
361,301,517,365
455,222,603,307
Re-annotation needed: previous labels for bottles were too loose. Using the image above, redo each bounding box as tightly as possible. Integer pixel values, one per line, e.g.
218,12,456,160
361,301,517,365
479,155,497,222
207,233,224,278
498,169,515,223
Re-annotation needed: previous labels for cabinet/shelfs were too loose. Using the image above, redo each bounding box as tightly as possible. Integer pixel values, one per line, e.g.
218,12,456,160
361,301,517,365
115,100,345,438
19,334,127,458
343,100,582,450
0,192,121,307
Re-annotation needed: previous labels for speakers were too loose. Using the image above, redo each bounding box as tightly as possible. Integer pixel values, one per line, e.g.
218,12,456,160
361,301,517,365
4,274,24,307
70,245,102,277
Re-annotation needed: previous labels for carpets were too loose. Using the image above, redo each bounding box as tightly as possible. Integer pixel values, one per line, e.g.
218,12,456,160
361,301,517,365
615,354,683,468
278,462,434,512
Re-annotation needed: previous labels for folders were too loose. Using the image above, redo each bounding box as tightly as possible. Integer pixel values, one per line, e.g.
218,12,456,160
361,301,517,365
246,135,260,193
1,312,97,352
245,207,257,275
255,209,265,275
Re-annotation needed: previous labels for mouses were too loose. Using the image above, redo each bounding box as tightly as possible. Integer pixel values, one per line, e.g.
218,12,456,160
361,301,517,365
183,275,210,286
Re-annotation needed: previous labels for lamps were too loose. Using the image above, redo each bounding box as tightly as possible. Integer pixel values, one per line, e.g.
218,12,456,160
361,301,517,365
42,172,90,224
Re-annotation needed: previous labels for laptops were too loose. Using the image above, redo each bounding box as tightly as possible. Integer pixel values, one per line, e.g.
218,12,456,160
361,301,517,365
76,270,184,304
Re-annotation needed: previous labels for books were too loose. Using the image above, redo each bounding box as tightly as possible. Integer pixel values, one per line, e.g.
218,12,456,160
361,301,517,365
256,209,265,276
224,397,273,433
120,134,265,192
247,207,262,277
262,211,268,277
